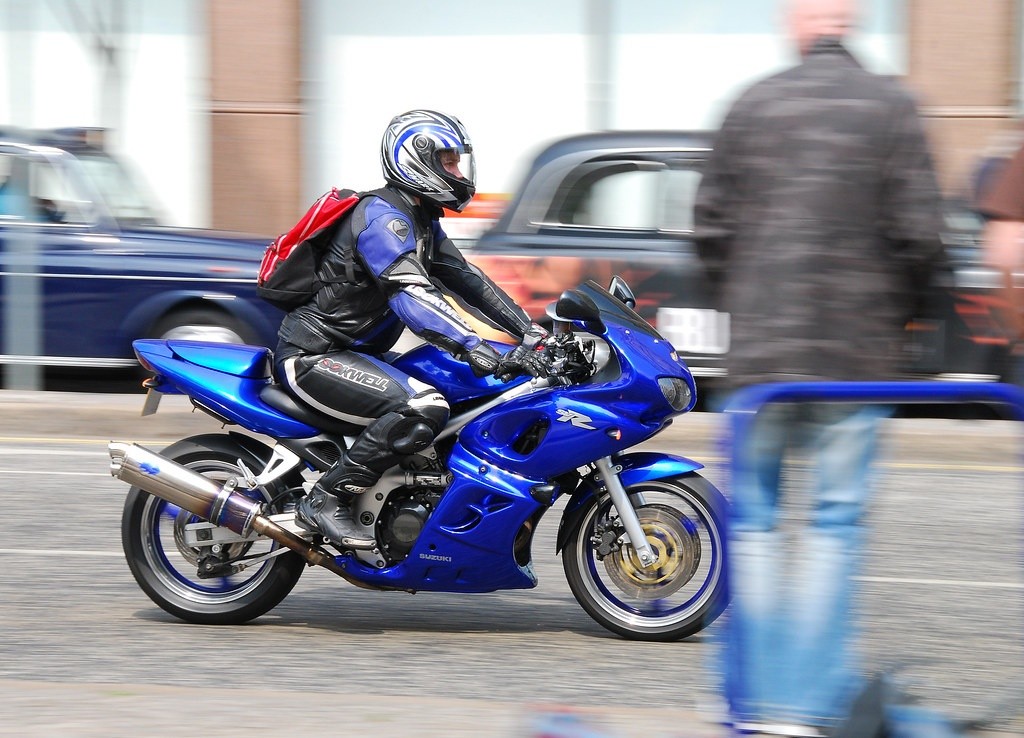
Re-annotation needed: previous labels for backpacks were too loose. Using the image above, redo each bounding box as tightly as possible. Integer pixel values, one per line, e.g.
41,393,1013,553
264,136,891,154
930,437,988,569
256,187,415,303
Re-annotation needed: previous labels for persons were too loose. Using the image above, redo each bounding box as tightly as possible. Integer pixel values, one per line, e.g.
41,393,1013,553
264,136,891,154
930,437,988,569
0,158,62,221
967,145,1024,269
694,0,955,738
272,109,553,550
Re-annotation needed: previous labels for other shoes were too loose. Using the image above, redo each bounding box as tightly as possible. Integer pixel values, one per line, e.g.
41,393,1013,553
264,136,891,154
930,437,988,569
718,700,821,736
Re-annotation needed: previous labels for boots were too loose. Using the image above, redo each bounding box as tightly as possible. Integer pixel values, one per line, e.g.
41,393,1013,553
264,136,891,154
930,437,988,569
295,449,378,549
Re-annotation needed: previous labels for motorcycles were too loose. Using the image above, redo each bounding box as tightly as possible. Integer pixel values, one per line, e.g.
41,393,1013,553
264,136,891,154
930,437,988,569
109,275,742,643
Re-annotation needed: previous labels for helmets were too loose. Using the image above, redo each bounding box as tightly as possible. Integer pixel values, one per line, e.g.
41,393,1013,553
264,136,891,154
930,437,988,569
381,109,475,213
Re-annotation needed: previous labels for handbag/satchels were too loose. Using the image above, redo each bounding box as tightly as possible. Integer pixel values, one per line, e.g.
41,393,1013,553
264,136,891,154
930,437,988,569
897,272,989,382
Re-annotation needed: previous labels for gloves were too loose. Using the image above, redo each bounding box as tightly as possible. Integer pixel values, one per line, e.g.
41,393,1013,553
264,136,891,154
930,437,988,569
539,335,555,346
493,345,553,380
